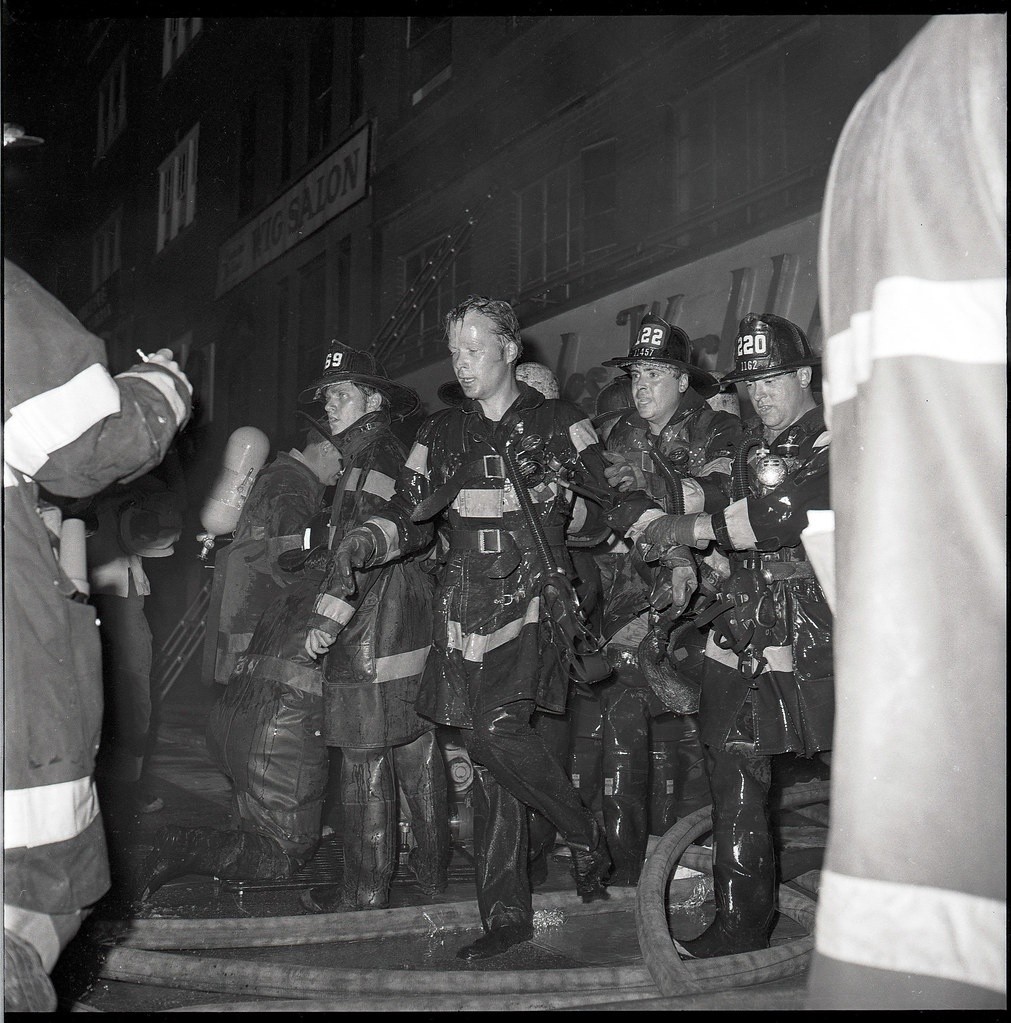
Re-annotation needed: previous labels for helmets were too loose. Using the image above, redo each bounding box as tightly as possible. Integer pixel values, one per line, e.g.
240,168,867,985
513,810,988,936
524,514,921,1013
295,408,343,453
119,500,181,557
277,508,333,572
601,312,720,399
722,313,821,382
590,374,637,429
298,348,421,423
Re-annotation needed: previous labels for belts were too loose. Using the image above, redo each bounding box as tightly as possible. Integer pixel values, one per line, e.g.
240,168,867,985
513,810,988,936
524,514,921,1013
447,529,565,579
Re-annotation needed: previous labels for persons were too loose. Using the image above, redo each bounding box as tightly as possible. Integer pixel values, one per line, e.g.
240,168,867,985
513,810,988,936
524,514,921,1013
5,256,198,1014
43,295,839,978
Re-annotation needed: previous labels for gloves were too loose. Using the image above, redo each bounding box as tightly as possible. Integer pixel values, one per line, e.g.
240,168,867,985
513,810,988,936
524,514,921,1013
336,530,374,595
648,544,698,619
601,450,667,500
636,511,710,561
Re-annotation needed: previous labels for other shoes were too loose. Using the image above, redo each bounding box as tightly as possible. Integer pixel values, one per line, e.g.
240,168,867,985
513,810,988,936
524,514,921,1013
101,783,165,812
408,846,448,895
134,825,193,900
574,828,613,896
300,886,367,914
672,922,763,959
458,924,532,961
